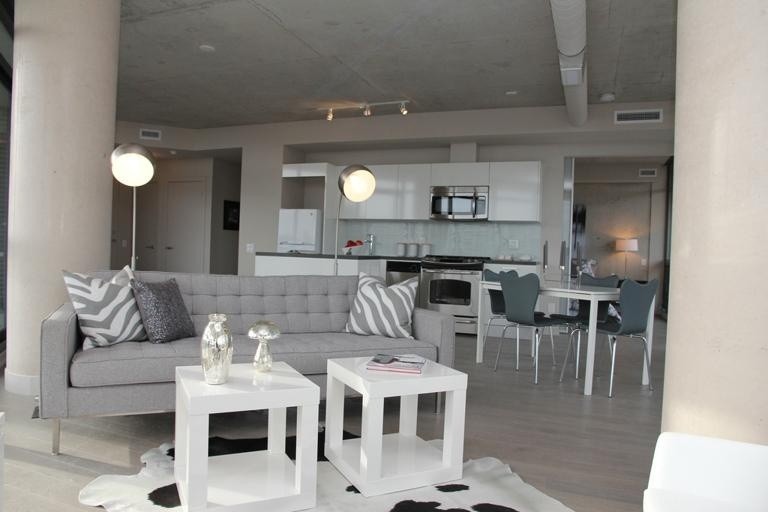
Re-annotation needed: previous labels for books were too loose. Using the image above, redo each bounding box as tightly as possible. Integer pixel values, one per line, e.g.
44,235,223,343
365,354,426,374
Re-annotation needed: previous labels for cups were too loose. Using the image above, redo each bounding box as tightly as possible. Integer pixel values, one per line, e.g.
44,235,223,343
397,243,406,257
407,243,419,257
420,243,430,258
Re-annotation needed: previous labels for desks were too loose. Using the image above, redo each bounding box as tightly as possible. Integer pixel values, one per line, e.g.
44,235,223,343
253,251,388,289
328,353,470,497
173,359,319,512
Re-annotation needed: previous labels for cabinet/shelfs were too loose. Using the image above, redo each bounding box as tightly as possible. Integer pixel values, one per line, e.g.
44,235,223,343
335,162,543,225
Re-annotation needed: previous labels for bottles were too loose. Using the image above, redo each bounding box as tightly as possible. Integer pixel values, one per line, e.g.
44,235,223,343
200,313,233,385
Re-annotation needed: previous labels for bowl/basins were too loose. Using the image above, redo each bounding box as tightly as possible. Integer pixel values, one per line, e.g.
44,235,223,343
495,255,513,261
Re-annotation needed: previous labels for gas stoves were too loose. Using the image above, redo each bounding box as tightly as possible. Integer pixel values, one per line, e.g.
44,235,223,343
422,254,490,269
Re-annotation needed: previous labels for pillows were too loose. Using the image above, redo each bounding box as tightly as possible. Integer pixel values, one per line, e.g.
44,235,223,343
341,273,421,342
126,278,195,344
61,260,147,351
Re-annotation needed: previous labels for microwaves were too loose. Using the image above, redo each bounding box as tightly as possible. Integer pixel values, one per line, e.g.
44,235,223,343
428,185,488,221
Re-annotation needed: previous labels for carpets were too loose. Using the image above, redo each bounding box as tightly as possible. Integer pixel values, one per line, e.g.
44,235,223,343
80,436,574,512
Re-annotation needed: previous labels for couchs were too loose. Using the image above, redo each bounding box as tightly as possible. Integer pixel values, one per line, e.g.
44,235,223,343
35,266,456,455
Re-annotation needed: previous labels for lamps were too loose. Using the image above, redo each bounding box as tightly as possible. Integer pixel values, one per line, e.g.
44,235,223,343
110,140,155,269
317,97,409,121
615,239,638,278
328,161,376,275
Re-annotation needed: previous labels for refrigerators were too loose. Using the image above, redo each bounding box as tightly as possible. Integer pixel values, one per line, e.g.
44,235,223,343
277,207,322,255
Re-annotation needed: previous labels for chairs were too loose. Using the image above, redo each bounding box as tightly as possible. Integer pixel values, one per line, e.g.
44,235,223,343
470,263,660,400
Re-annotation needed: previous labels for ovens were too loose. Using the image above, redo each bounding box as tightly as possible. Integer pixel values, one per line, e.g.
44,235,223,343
420,266,482,337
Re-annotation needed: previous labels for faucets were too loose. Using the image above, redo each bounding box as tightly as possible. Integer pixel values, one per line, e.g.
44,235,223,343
364,233,375,256
